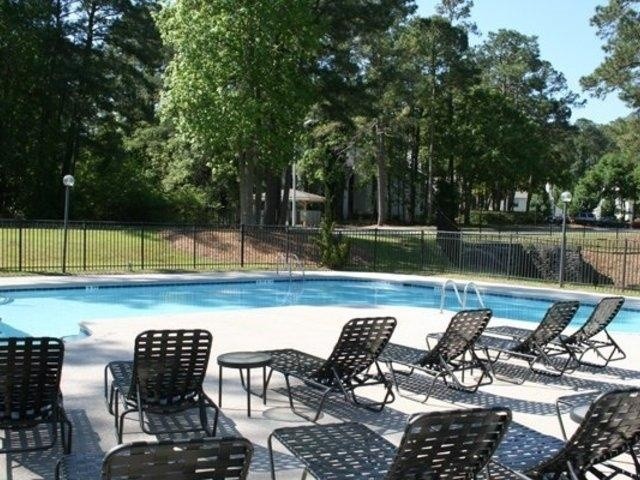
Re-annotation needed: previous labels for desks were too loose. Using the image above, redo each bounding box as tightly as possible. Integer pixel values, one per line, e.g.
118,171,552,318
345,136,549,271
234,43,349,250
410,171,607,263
216,350,275,418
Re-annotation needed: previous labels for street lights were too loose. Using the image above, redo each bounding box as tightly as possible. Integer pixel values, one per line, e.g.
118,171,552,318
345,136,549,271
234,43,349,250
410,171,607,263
558,191,573,290
60,175,75,276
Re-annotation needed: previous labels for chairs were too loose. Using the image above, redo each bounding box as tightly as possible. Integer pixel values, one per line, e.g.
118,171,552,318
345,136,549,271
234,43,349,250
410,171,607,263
104,329,221,443
427,301,579,384
1,338,71,454
485,296,625,366
55,437,255,480
555,391,640,480
255,317,396,423
267,407,512,480
363,310,492,403
430,387,640,480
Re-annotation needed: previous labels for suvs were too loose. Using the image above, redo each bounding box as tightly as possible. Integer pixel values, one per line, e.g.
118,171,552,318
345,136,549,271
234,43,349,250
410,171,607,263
573,213,595,225
